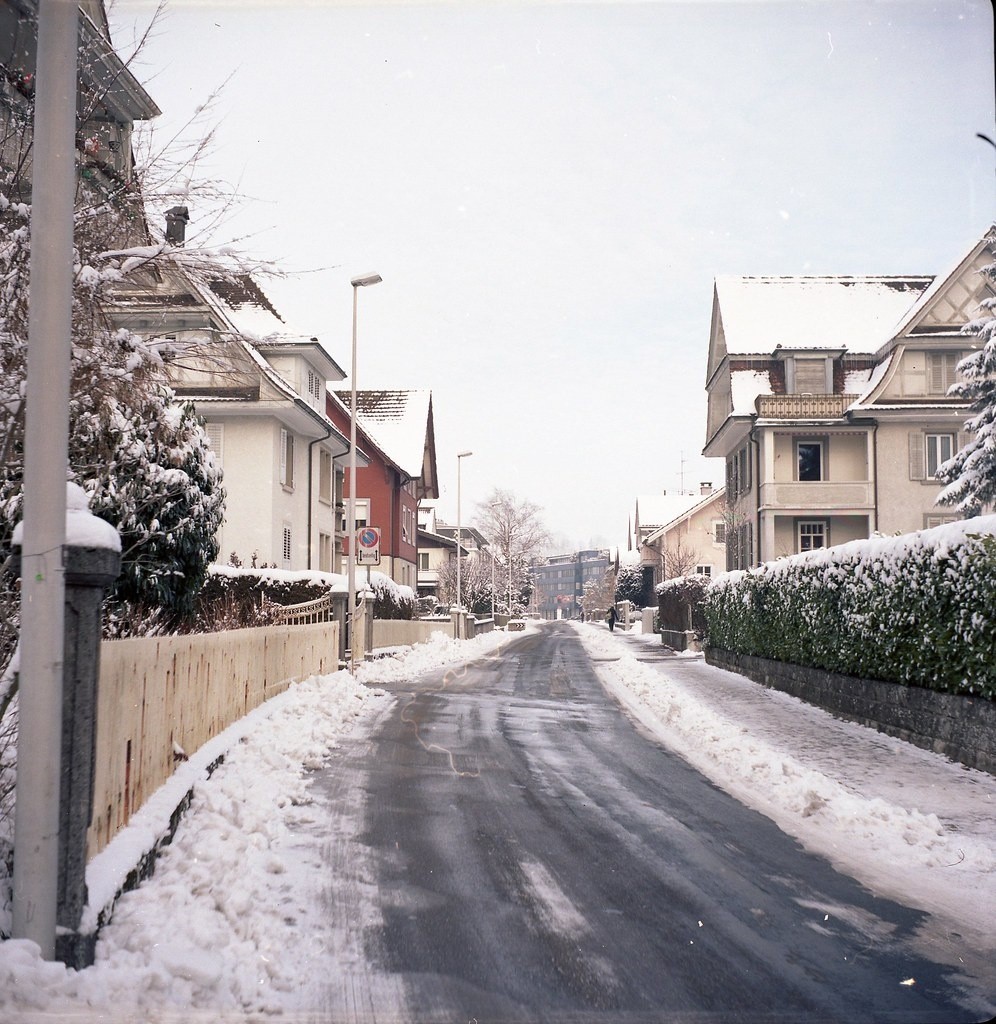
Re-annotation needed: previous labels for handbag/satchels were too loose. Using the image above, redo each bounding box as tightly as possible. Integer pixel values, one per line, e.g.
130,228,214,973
605,609,612,619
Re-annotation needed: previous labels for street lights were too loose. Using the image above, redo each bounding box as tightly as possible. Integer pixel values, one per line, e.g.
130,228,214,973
491,501,504,621
453,450,475,606
344,274,387,675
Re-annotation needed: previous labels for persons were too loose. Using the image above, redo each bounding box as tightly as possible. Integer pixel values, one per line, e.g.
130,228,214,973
581,612,584,623
607,606,618,632
